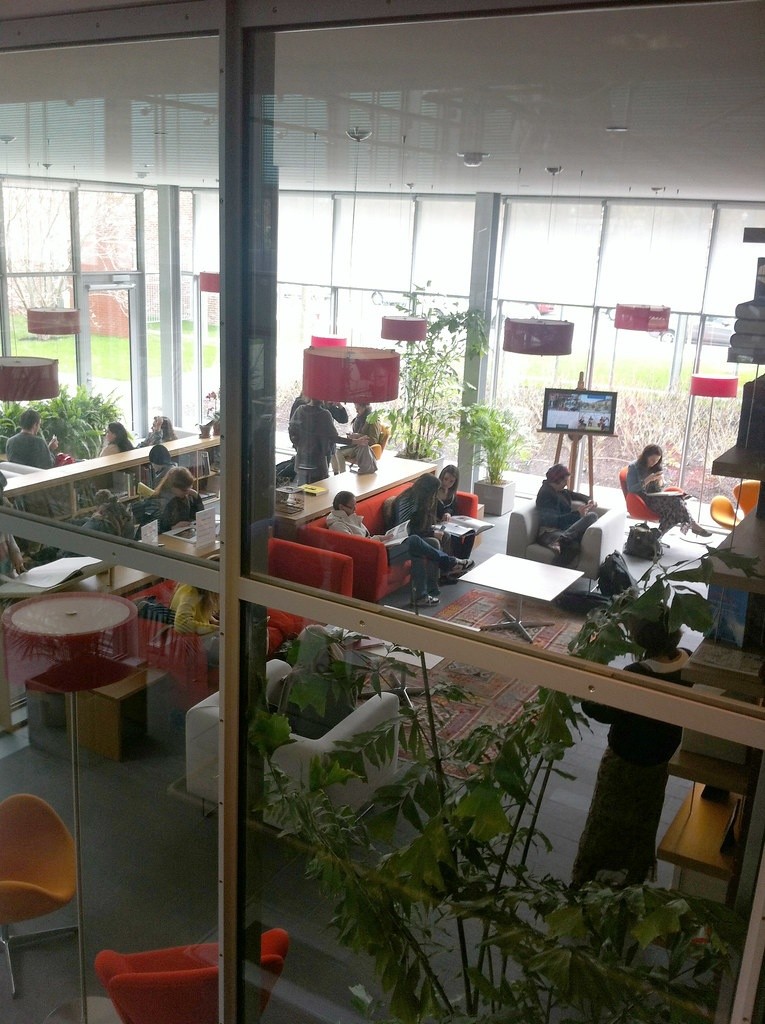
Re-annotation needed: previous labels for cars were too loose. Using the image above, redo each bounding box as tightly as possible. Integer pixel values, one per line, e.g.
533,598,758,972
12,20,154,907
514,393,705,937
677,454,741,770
650,308,736,347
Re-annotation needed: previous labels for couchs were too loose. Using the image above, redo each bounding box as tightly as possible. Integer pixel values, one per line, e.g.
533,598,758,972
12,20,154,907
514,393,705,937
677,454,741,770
134,541,356,710
184,660,398,842
507,498,615,580
299,480,480,603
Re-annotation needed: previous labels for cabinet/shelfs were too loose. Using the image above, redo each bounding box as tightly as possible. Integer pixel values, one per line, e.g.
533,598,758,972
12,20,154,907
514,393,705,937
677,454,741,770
3,433,222,564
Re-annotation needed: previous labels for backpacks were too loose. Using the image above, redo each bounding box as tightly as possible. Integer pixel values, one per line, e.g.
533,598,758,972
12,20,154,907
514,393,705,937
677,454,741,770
598,551,639,598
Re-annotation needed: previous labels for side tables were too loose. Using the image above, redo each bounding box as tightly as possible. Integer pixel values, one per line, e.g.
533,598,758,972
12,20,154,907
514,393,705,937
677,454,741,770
65,677,148,760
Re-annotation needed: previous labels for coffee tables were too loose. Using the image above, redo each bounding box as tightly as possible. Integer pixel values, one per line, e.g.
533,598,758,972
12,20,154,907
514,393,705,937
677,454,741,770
456,552,582,645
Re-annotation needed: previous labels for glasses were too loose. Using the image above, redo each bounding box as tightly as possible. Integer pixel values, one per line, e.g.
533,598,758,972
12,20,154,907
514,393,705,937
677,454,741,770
343,503,358,509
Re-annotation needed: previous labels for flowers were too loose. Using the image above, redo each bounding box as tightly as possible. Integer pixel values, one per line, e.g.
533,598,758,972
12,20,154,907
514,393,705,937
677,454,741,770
207,391,221,416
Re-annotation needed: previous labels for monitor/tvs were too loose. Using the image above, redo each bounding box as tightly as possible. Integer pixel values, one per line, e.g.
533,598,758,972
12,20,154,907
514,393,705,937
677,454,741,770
542,388,617,435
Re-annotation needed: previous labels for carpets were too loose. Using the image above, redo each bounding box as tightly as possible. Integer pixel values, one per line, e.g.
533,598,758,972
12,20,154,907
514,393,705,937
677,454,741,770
357,589,588,791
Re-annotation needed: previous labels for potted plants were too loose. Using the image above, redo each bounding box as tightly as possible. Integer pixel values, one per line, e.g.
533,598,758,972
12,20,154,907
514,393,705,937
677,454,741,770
466,399,530,514
370,282,490,478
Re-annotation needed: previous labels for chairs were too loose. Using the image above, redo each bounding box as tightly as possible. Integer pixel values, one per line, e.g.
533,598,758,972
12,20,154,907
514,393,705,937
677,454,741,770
619,464,686,545
1,790,73,997
710,481,760,531
94,926,289,1024
349,423,390,473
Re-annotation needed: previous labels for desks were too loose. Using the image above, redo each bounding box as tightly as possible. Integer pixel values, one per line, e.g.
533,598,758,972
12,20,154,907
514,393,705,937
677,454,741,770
656,790,747,905
273,456,437,538
46,518,223,599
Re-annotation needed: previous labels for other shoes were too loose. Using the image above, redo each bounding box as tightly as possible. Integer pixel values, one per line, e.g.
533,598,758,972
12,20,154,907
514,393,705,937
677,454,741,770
441,575,459,584
559,537,581,554
692,528,712,537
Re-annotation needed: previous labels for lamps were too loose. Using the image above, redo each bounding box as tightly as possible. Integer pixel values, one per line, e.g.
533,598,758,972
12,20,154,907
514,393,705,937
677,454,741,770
312,195,348,348
0,592,138,1023
688,320,738,397
303,129,398,401
502,167,573,355
0,235,60,401
199,177,222,293
614,187,670,330
379,192,431,342
28,163,80,334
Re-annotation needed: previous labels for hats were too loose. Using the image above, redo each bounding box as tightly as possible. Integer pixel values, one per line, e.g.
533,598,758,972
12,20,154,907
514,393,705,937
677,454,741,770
149,445,171,465
546,464,568,482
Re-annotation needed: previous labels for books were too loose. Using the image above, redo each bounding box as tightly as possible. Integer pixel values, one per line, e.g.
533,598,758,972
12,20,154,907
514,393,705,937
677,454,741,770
432,515,495,538
199,451,211,476
703,583,749,649
0,557,103,588
385,520,410,546
298,483,328,497
75,465,154,511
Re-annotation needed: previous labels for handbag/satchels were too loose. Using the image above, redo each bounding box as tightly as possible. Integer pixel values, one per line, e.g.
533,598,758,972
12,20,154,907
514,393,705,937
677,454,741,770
565,592,613,612
623,523,664,563
349,444,378,474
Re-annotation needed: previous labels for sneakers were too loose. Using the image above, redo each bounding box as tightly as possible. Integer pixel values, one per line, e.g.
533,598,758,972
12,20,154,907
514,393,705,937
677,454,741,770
453,558,475,571
411,595,439,606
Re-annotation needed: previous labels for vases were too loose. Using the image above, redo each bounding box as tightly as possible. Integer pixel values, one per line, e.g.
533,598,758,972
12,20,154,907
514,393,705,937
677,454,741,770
213,417,224,434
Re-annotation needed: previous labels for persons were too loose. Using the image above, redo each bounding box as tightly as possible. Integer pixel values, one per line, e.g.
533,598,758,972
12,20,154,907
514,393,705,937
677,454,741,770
169,554,219,666
100,423,133,457
133,445,204,535
289,390,381,484
0,472,28,578
392,464,475,594
580,415,609,431
570,603,696,893
327,491,476,607
536,464,598,568
6,409,58,470
267,625,354,740
56,490,134,558
627,444,712,540
137,416,178,449
